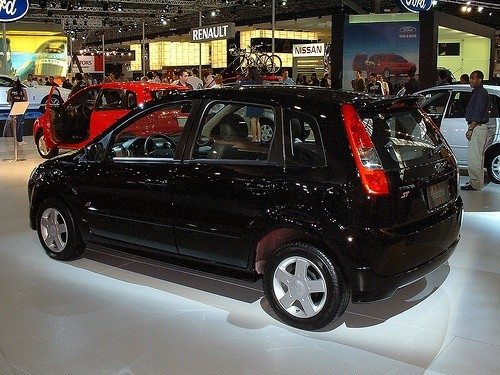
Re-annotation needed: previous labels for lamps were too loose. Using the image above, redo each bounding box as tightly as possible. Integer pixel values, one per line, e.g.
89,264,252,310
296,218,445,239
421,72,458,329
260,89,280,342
39,0,182,27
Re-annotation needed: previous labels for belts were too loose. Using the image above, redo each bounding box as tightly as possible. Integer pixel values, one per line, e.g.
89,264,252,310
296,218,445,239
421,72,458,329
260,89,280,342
468,121,486,124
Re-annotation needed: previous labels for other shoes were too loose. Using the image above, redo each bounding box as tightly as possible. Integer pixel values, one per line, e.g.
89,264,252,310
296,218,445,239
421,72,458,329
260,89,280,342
19,141,23,145
460,184,477,190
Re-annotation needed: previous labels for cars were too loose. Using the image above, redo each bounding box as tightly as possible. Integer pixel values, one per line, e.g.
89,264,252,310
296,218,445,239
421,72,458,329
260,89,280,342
0,74,72,137
203,82,312,144
387,83,500,185
365,53,417,79
26,82,465,332
33,82,208,159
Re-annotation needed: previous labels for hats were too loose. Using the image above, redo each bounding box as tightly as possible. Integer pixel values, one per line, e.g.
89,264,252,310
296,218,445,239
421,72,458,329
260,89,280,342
75,73,83,80
14,76,21,81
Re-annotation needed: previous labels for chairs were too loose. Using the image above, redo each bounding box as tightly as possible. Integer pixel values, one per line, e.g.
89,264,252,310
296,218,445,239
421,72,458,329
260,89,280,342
258,119,303,158
102,91,122,110
455,98,466,115
206,113,250,159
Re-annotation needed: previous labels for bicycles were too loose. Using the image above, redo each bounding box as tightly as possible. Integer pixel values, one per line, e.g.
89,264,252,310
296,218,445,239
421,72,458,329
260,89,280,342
229,42,282,75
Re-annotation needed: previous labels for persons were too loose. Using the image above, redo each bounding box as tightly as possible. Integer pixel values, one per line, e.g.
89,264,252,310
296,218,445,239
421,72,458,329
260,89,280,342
240,66,263,84
26,67,215,90
460,70,489,191
7,78,28,142
280,69,330,87
353,69,469,96
